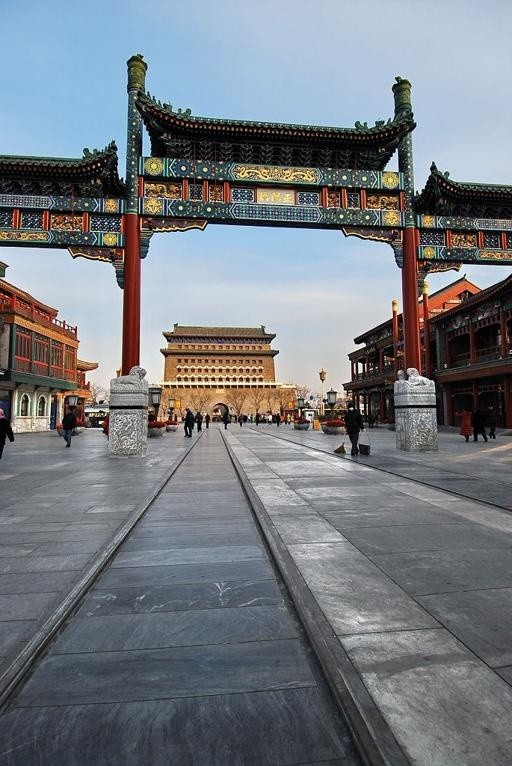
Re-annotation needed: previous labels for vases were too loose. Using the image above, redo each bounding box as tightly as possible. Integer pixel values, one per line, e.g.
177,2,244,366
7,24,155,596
56,428,87,436
293,422,310,431
321,424,345,435
148,425,178,439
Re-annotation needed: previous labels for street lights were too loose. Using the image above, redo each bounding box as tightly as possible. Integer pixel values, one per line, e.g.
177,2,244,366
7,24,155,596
68,392,79,436
150,389,176,422
319,368,328,415
326,388,337,420
297,396,305,419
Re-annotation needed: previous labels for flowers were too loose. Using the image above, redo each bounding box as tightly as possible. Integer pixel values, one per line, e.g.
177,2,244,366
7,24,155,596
297,418,310,423
148,420,177,428
56,416,86,429
326,420,345,427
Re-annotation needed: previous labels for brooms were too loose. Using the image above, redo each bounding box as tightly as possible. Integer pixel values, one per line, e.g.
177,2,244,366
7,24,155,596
334,431,346,453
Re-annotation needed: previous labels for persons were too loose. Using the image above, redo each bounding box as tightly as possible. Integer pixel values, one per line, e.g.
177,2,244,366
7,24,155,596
343,403,366,457
486,405,498,439
223,409,231,429
205,413,212,428
213,412,291,427
470,407,488,443
454,408,473,442
0,408,15,461
182,408,195,438
194,411,203,433
147,412,156,423
61,408,76,448
